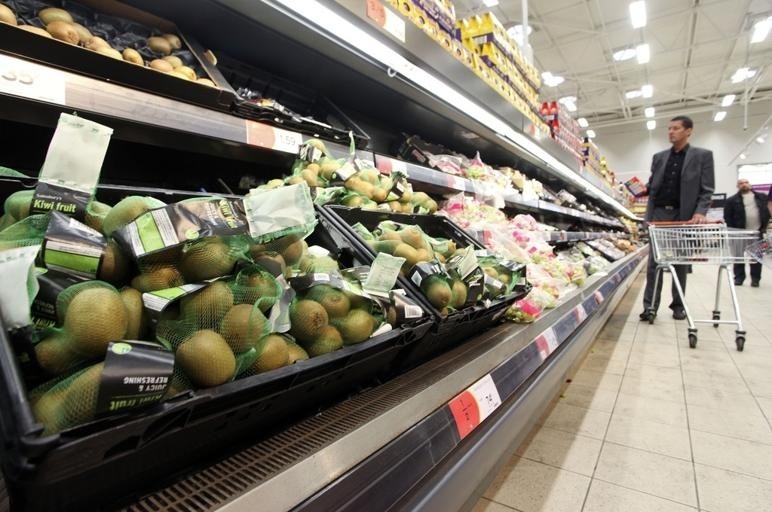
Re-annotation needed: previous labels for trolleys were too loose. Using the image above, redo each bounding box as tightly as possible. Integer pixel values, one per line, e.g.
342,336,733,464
644,218,760,352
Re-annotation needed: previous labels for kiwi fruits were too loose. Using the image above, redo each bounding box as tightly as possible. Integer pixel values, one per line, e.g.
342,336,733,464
0,182,425,443
348,217,528,319
0,0,216,90
244,138,441,216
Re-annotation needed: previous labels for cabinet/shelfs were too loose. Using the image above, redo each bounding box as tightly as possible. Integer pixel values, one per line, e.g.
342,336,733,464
1,1,657,511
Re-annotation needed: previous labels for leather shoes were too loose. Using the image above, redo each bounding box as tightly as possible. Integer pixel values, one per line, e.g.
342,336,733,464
673,312,684,319
640,311,657,319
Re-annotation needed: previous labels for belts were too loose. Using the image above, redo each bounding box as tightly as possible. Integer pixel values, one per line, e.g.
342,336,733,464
658,205,679,210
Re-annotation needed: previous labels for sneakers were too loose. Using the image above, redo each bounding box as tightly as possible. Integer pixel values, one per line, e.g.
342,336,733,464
735,280,742,285
751,281,759,286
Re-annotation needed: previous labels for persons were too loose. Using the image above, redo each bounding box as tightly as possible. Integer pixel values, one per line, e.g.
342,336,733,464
765,185,772,223
722,178,767,287
635,115,716,322
